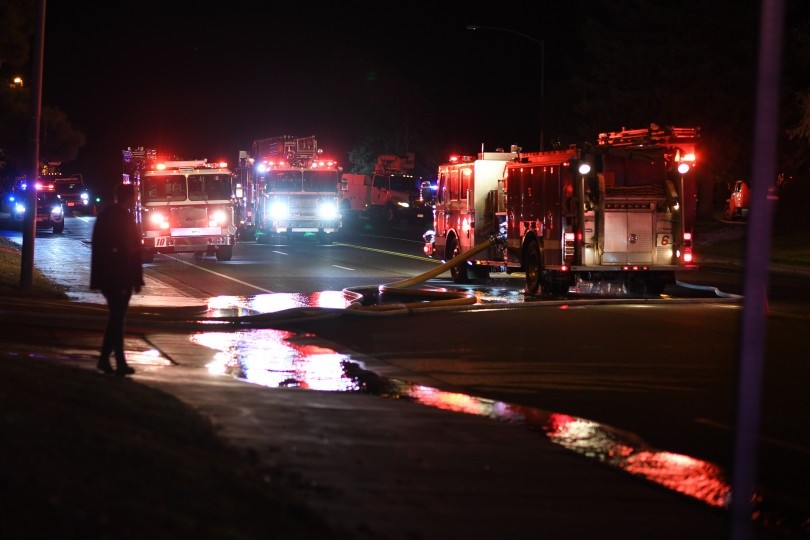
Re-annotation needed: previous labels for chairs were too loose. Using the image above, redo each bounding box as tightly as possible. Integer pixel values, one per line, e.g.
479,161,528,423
206,180,224,194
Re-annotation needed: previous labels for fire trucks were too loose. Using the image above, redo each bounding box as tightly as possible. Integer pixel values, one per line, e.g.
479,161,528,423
338,154,426,223
126,157,245,266
233,135,349,247
420,123,705,298
39,161,93,217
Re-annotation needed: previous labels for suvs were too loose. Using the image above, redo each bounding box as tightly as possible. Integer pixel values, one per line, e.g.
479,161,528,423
725,174,782,223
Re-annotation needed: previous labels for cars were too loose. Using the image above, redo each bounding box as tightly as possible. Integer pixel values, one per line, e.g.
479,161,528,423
8,180,68,234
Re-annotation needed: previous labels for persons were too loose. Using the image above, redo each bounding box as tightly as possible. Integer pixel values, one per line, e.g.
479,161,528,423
87,184,146,374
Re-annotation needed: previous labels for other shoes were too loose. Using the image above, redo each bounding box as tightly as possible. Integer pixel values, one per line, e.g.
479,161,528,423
97,356,114,374
116,363,135,375
